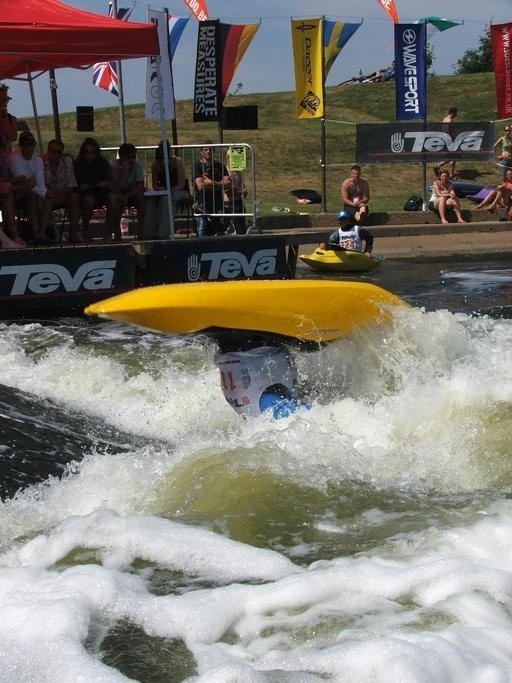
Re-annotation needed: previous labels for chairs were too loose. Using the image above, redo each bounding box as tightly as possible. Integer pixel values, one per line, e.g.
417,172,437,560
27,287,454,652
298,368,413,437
173,176,198,238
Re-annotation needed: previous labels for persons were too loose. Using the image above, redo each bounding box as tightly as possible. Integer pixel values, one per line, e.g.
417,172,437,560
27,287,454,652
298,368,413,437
220,148,250,237
6,130,51,242
206,335,312,422
341,165,370,223
41,139,85,243
432,106,459,178
72,138,121,242
110,142,146,239
1,181,28,249
322,212,374,255
1,83,28,249
471,169,512,210
362,59,397,84
192,140,232,236
492,124,512,182
428,169,468,225
145,139,190,239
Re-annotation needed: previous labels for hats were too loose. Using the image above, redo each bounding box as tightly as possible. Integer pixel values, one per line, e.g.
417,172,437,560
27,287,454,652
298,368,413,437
19,131,36,147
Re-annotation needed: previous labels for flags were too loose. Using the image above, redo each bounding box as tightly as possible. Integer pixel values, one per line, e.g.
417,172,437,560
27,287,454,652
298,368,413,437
220,22,260,106
166,12,188,64
93,1,120,100
319,19,362,83
414,15,459,33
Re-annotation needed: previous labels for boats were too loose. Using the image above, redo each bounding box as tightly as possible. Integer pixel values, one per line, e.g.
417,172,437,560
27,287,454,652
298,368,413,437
471,186,499,205
426,178,484,194
299,236,390,271
84,280,416,345
0,135,308,304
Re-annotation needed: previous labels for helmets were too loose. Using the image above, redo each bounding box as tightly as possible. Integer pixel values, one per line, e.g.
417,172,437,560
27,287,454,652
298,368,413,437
336,211,349,220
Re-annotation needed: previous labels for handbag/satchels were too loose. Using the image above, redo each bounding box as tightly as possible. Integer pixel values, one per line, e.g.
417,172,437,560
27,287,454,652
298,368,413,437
404,196,423,211
